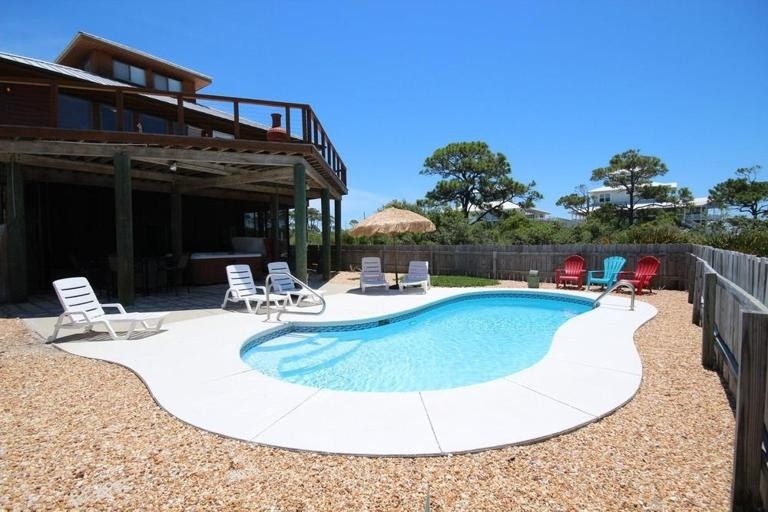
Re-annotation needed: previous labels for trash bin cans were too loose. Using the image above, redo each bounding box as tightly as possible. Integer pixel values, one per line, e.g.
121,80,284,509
527,270,540,288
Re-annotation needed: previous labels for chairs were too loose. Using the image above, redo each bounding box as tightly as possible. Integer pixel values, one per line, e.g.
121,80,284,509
359,257,431,294
555,254,661,295
221,261,328,315
52,276,170,341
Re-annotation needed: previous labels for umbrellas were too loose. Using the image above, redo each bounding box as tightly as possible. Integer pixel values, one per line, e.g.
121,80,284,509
348,204,436,285
